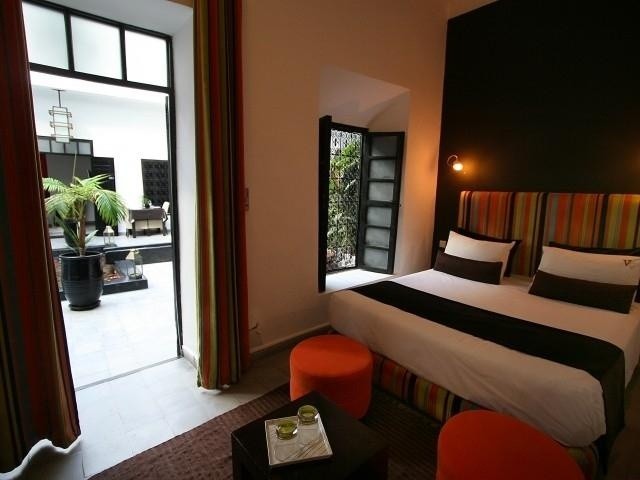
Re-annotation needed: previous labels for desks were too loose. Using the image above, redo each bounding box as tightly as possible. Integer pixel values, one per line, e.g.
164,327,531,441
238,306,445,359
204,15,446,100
126,206,168,238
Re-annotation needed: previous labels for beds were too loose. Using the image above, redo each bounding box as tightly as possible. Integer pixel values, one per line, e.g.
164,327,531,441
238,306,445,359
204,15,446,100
328,191,640,473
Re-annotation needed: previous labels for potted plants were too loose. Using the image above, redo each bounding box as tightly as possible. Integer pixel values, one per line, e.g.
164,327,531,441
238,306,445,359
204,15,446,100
42,172,126,311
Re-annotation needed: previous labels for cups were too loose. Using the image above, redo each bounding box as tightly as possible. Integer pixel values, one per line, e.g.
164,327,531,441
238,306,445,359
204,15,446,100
273,422,299,461
297,405,320,446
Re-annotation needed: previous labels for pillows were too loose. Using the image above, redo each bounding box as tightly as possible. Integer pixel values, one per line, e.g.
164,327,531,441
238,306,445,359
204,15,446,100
433,237,516,285
457,228,521,278
527,246,640,316
548,241,640,304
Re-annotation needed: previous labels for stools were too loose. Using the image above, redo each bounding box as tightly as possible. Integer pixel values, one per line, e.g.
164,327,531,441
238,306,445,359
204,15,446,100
289,335,373,419
436,409,585,480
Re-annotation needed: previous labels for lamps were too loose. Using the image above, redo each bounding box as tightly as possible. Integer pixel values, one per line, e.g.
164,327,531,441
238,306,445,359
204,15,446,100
47,87,74,144
446,154,462,171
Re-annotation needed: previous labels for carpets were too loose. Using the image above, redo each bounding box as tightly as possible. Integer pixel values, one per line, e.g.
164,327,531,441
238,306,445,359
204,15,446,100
86,382,442,480
